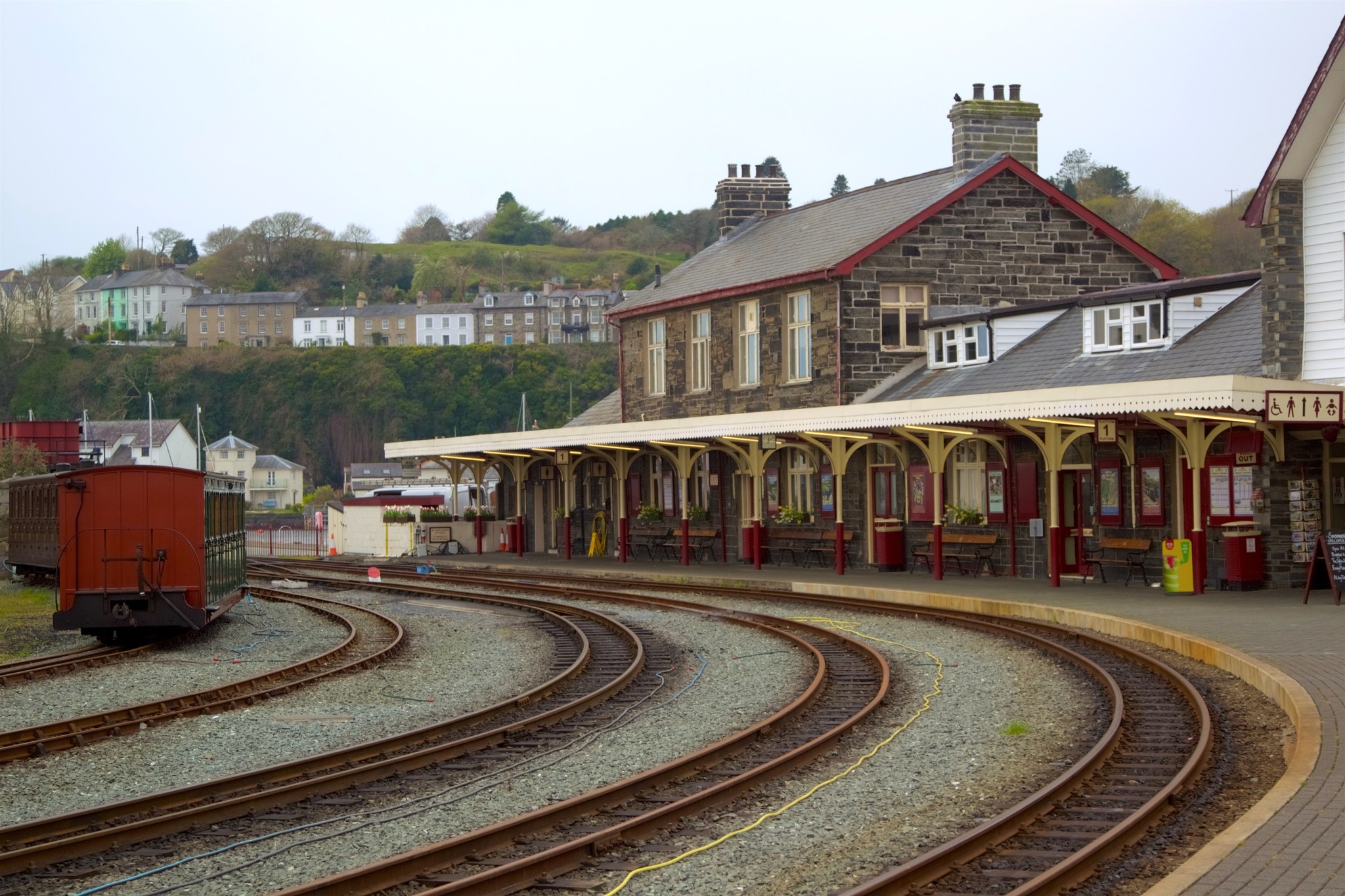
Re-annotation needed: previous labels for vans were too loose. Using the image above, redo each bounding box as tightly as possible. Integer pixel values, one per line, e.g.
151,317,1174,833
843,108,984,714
361,483,489,520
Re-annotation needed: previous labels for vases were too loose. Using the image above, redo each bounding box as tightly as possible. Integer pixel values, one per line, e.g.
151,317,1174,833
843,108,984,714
962,513,983,525
465,515,496,521
793,513,809,524
421,516,453,523
382,516,396,524
694,511,711,521
396,515,416,523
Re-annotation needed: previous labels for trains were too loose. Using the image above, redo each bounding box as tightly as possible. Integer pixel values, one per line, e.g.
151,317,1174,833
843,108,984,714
6,464,248,643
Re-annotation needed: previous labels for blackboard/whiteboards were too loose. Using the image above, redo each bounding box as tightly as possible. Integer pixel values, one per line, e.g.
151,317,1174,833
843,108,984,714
1307,528,1345,593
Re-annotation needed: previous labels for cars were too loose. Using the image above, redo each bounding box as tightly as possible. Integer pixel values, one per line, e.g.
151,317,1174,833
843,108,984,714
78,340,91,346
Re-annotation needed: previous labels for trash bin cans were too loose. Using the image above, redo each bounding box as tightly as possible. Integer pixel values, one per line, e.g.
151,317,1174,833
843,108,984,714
505,516,522,553
741,517,765,565
1221,520,1264,591
873,517,906,573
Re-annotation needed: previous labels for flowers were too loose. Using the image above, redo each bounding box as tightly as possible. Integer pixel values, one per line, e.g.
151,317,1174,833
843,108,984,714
686,500,706,521
420,506,451,517
384,504,411,517
550,508,565,522
941,499,985,529
769,506,806,524
464,504,493,516
635,500,659,527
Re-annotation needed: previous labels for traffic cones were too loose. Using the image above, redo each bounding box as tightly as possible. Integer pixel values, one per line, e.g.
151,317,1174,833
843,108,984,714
503,533,510,552
327,533,338,556
496,528,506,552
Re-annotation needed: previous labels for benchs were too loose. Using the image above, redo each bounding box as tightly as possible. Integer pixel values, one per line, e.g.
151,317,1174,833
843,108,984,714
909,533,999,577
617,528,670,560
1081,538,1151,587
659,528,718,563
803,531,855,569
761,528,822,567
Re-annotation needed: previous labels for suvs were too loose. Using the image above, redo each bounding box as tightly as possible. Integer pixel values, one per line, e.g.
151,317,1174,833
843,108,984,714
104,340,125,347
